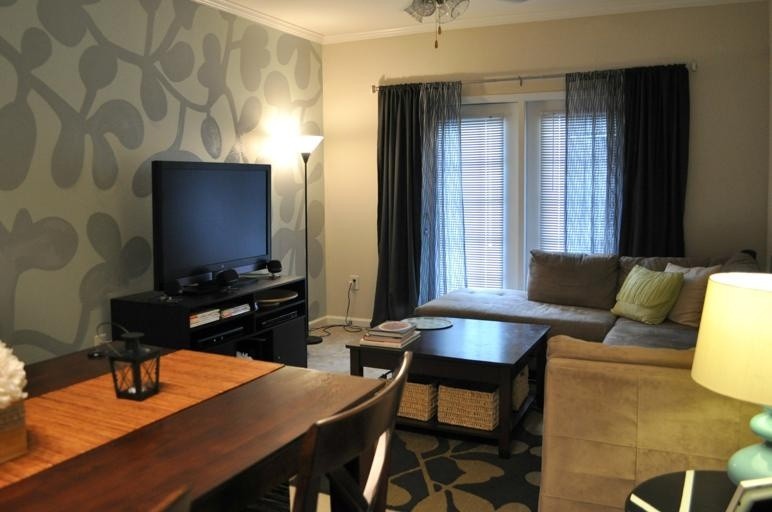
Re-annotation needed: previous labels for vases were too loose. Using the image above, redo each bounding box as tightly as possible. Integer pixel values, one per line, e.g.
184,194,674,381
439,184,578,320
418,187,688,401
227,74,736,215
1,400,29,464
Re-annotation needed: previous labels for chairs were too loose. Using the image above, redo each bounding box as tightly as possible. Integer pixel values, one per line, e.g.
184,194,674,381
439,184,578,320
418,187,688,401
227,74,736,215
204,350,415,511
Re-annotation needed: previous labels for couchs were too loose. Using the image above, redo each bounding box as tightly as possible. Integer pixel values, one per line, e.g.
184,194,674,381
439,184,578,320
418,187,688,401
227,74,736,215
413,247,765,511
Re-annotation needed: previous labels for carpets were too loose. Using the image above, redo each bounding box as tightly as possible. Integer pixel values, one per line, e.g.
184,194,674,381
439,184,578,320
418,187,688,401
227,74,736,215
285,384,548,512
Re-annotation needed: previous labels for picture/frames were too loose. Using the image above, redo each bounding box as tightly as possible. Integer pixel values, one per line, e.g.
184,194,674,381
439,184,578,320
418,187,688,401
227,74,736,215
724,477,772,512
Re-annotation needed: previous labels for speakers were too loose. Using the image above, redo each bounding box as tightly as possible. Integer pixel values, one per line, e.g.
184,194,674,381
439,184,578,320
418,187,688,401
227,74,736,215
164,279,184,303
268,261,281,280
217,270,240,294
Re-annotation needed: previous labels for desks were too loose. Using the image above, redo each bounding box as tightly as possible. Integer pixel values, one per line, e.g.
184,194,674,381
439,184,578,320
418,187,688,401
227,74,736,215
0,338,387,512
623,469,738,512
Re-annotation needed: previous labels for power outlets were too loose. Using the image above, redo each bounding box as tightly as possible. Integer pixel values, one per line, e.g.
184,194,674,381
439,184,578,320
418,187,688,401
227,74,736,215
348,275,359,290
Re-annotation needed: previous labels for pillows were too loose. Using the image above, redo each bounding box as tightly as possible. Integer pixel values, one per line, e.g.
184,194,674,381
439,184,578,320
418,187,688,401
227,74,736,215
609,264,685,326
663,261,723,328
720,251,761,272
527,248,618,310
618,256,730,293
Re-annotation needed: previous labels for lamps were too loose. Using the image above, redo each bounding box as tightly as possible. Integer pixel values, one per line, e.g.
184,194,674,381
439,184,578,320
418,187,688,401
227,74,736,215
294,135,326,344
689,271,772,486
403,0,470,25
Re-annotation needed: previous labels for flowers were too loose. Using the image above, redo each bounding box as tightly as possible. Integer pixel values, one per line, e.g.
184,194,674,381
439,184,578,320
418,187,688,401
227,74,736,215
0,339,30,410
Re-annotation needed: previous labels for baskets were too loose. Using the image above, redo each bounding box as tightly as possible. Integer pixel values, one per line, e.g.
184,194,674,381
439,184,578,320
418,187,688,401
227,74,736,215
437,365,530,431
380,370,438,422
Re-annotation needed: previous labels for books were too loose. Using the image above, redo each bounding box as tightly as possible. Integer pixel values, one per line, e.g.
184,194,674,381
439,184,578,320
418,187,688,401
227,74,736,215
359,319,422,349
189,302,251,329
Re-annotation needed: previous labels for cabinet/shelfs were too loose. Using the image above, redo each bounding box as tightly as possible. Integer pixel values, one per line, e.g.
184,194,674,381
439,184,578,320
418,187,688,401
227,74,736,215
111,275,308,367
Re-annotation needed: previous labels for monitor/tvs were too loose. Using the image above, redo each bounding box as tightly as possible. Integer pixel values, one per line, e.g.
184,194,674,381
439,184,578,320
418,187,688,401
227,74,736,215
152,160,271,295
